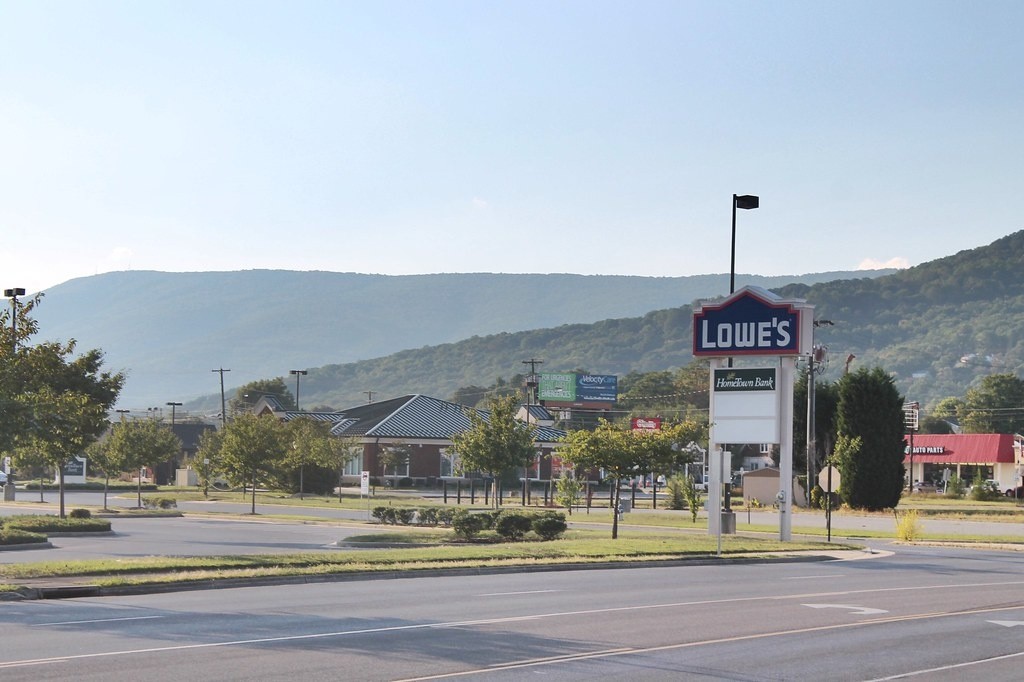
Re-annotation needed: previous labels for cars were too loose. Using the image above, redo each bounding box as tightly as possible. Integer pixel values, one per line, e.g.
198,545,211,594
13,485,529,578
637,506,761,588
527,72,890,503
912,481,942,493
729,474,742,488
0,471,9,486
970,478,995,491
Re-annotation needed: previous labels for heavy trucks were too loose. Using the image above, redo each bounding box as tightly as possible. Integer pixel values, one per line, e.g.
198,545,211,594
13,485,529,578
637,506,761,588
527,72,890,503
991,462,1024,497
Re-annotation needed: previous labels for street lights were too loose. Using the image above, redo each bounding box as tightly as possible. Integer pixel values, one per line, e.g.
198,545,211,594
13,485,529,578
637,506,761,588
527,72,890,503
289,369,307,410
116,408,130,418
4,287,26,339
166,402,183,433
721,192,760,533
